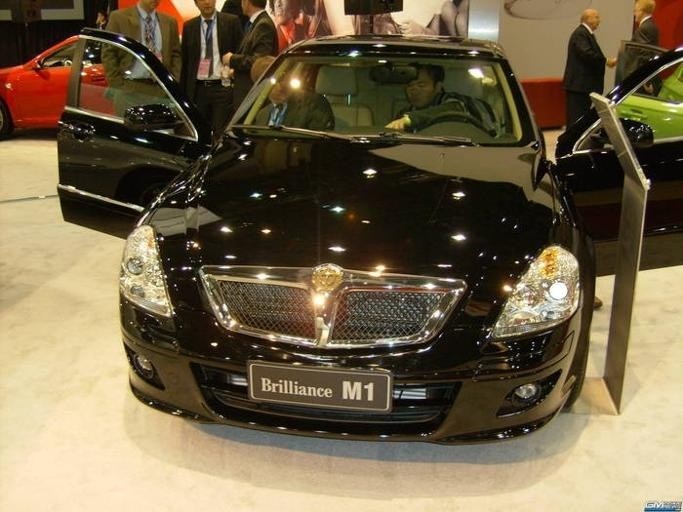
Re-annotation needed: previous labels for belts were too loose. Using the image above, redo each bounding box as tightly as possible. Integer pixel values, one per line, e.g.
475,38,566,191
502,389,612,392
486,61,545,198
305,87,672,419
196,79,221,88
128,77,159,85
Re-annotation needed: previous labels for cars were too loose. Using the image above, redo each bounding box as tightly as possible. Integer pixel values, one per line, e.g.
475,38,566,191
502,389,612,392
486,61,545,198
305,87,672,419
0,27,683,441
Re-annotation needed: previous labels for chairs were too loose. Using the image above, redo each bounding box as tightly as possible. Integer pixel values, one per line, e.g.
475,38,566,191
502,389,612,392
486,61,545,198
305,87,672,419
313,63,506,137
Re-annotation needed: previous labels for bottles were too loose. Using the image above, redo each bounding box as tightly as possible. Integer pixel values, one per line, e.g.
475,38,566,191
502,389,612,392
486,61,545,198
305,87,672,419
221,63,230,87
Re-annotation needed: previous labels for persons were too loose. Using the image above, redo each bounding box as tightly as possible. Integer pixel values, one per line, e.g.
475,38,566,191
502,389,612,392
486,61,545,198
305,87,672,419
439,1,470,36
266,1,335,50
559,5,617,131
249,58,335,130
221,1,278,114
105,1,179,128
610,1,661,97
172,0,244,156
381,62,504,141
348,9,442,39
95,11,108,60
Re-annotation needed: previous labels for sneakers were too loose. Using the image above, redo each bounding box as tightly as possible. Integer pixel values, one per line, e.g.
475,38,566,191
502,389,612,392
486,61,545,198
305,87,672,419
592,296,604,310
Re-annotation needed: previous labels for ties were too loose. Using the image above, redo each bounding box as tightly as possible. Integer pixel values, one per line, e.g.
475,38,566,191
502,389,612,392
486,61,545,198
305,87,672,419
244,20,254,33
203,19,214,78
268,102,286,125
143,14,158,83
